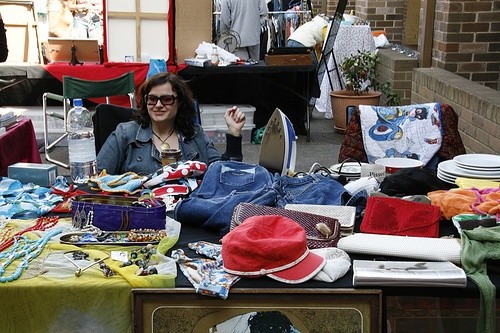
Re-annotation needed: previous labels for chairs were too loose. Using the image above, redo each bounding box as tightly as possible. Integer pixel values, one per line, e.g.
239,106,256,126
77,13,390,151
344,103,460,172
92,98,201,160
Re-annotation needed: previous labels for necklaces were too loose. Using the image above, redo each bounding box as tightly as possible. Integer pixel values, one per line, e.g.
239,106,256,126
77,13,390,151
152,129,175,150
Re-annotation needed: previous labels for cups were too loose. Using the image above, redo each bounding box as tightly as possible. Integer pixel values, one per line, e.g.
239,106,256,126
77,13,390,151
161,148,183,167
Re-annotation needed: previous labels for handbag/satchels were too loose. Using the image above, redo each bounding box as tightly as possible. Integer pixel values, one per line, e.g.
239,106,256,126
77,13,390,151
230,202,341,249
284,204,356,238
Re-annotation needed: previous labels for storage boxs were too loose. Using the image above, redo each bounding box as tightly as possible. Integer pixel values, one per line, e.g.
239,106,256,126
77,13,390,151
7,162,58,188
265,53,313,66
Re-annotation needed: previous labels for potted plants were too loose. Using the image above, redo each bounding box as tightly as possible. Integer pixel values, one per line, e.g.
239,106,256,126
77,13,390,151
329,48,402,135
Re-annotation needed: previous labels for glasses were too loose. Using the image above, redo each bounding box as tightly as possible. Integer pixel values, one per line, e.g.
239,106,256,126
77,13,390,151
144,95,178,105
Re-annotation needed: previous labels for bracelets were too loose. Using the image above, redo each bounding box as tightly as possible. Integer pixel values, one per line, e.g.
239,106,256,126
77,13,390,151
128,226,166,243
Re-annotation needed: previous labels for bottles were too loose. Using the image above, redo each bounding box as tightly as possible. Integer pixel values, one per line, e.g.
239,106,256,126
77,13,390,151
211,47,219,65
67,98,99,184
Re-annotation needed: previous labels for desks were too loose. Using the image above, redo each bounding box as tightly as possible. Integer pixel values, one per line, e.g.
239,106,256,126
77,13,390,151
0,204,500,333
45,60,150,109
178,58,317,135
0,117,43,177
0,61,56,107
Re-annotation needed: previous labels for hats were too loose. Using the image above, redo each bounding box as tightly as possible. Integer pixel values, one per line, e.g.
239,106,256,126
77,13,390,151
222,215,327,284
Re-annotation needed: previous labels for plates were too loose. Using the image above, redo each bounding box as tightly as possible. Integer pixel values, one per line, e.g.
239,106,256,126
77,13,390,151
437,154,500,185
330,162,369,179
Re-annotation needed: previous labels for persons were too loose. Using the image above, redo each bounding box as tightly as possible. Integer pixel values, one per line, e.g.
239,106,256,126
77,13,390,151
96,73,245,176
287,16,329,47
220,0,268,61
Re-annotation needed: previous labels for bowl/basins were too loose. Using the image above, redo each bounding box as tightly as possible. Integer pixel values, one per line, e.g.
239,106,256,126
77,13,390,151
375,158,423,173
